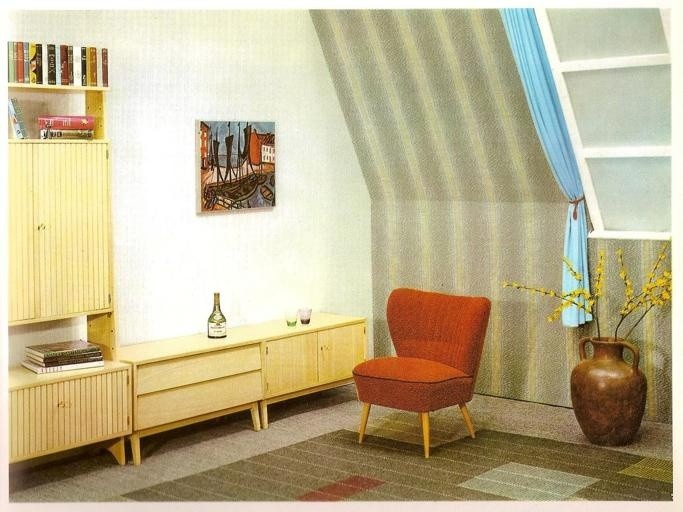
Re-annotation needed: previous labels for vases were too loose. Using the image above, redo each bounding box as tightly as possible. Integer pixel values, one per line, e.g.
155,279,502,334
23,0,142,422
570,339,647,447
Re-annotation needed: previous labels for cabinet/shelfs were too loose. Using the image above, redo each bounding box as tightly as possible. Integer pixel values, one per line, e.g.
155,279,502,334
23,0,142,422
118,313,367,465
8,81,133,466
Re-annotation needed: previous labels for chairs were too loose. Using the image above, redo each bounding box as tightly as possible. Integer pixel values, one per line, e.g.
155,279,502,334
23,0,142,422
352,289,491,458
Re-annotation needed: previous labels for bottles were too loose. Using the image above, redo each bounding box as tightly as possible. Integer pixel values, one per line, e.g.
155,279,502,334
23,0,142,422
208,290,228,339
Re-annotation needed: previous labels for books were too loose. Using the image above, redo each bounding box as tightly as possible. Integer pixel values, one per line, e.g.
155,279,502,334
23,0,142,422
8,99,23,141
10,97,28,140
19,358,104,374
33,115,94,130
34,128,94,142
25,349,103,362
7,40,110,85
25,355,103,368
24,338,100,359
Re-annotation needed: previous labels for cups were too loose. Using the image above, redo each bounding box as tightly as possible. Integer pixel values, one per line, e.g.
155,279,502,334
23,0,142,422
299,307,313,325
282,307,299,328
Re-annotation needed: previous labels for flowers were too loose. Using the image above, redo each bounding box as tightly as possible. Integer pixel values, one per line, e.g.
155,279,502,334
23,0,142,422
500,235,672,340
